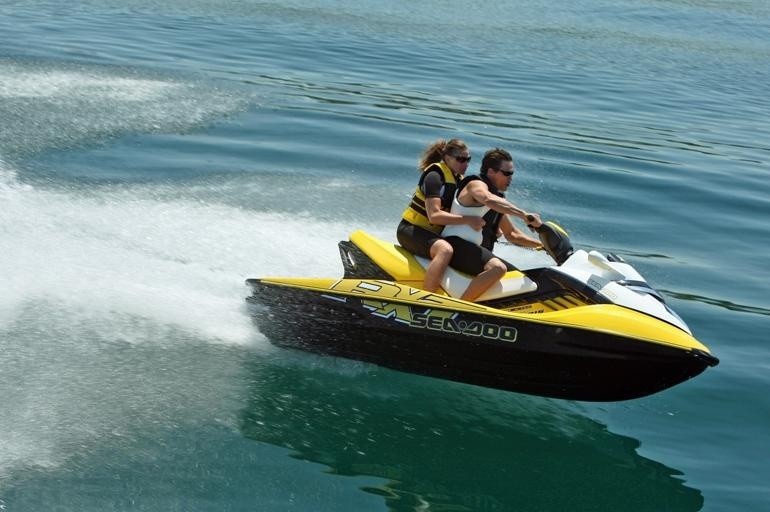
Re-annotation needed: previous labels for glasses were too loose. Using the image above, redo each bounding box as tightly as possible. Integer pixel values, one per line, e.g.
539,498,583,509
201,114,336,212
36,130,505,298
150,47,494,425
449,154,471,162
496,169,514,176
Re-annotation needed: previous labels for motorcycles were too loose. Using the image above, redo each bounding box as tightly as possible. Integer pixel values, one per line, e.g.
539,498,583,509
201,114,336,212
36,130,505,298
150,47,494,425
245,214,720,402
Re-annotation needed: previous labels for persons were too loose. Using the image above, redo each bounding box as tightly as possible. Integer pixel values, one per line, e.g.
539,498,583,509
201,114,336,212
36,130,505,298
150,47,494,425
396,138,503,294
440,150,544,302
